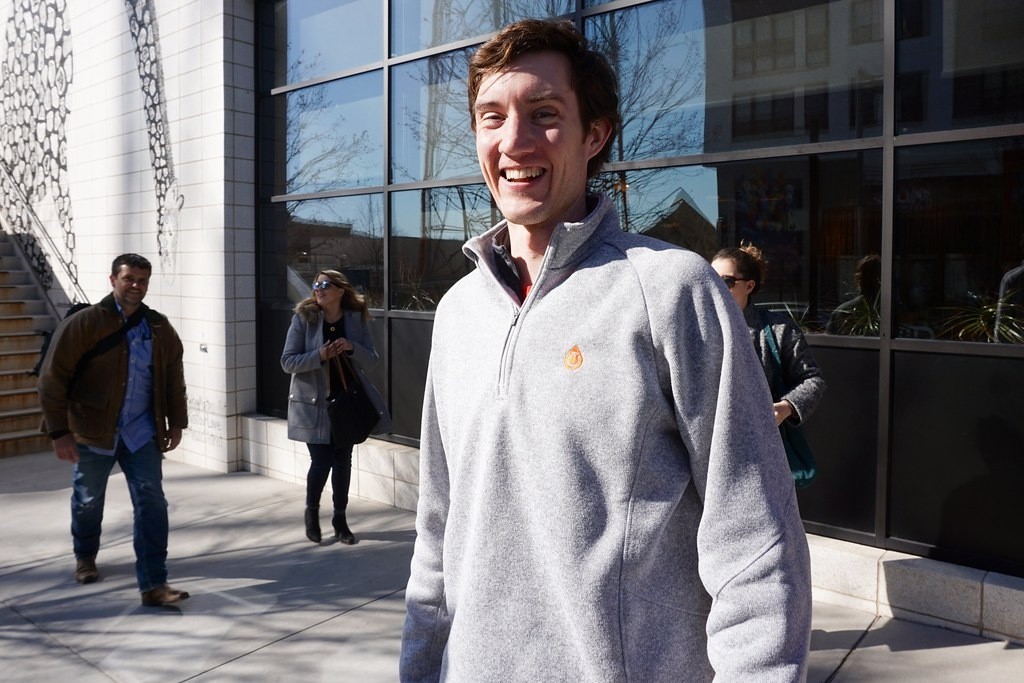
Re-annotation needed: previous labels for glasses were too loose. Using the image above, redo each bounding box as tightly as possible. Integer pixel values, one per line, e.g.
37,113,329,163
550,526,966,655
722,275,749,290
312,281,335,290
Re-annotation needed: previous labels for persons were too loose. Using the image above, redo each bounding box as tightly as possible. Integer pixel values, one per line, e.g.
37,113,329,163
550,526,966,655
281,270,395,545
396,16,815,683
38,253,192,606
710,239,825,428
828,255,882,330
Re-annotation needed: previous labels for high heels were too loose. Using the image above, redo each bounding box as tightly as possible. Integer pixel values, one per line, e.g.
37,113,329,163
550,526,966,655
305,510,321,543
332,517,354,544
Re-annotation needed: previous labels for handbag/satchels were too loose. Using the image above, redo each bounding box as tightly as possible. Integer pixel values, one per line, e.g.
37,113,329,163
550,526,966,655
760,309,818,489
326,342,381,449
26,303,92,377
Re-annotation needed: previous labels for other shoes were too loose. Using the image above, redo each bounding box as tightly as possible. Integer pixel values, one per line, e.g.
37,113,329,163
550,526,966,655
76,555,99,583
142,585,189,607
